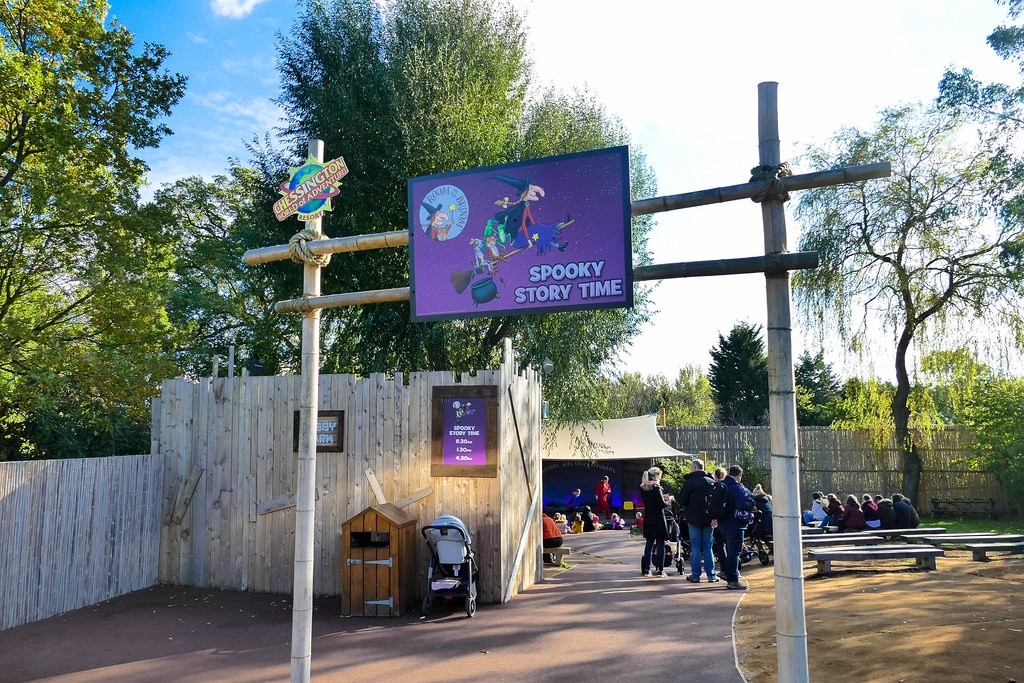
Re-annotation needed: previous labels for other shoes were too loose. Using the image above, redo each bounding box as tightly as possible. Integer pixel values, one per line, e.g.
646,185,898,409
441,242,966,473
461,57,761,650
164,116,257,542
708,576,720,583
645,572,653,577
717,571,728,581
658,571,668,578
727,580,748,590
686,576,700,583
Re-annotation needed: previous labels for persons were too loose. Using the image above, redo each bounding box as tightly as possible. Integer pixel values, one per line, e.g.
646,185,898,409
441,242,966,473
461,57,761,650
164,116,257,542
639,466,675,578
834,494,867,533
750,483,773,556
714,466,741,580
562,487,583,526
817,491,830,507
889,494,914,541
898,494,920,529
816,492,845,530
542,513,564,566
551,504,645,535
801,492,830,526
593,475,612,520
717,464,749,590
860,494,882,531
873,494,897,542
678,458,720,583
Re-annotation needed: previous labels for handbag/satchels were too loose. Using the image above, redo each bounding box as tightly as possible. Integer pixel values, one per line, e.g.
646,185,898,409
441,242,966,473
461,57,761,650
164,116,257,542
734,508,755,524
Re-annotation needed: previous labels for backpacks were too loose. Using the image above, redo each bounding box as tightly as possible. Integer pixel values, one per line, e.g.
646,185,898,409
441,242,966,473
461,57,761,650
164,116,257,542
704,481,741,520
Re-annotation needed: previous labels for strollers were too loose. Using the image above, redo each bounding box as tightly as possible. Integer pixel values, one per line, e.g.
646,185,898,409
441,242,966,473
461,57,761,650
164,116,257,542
652,508,686,575
737,510,771,570
420,514,481,618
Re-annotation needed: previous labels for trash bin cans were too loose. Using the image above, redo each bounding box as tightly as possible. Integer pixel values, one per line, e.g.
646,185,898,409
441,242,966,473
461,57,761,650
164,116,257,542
341,502,418,618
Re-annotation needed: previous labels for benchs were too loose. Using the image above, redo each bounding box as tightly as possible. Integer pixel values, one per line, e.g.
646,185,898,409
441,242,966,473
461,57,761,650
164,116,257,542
800,520,1024,579
541,546,572,566
